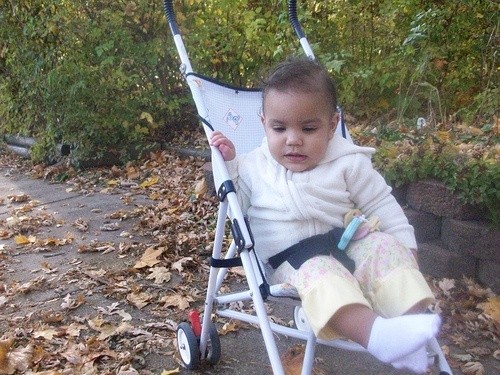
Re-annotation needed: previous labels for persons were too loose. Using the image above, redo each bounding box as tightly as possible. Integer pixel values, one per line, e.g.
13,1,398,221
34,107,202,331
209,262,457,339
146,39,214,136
209,56,443,374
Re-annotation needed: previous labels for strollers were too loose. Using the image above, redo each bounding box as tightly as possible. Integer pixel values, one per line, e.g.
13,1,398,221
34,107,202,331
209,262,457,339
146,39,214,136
162,0,453,375
